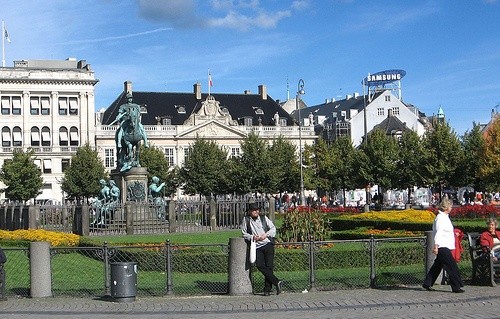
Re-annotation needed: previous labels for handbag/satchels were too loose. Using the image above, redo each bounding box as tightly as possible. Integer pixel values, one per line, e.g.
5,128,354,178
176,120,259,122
249,235,256,263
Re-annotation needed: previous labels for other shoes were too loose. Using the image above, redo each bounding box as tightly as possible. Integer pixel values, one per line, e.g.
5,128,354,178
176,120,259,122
264,291,269,296
422,280,435,290
452,288,464,293
276,280,284,295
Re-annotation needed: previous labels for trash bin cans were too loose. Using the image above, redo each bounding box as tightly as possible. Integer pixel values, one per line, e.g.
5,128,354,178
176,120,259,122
110,261,139,303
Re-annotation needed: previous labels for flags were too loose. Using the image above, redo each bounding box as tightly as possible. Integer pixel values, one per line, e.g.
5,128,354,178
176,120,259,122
209,73,212,86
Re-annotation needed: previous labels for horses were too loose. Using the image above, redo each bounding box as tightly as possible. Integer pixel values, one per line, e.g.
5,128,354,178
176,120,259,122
115,107,144,167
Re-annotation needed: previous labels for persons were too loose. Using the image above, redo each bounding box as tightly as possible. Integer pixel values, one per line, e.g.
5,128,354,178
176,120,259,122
90,179,120,225
373,192,384,205
274,193,334,212
422,197,465,293
463,189,500,205
117,94,149,149
480,218,500,259
241,203,282,296
148,177,166,218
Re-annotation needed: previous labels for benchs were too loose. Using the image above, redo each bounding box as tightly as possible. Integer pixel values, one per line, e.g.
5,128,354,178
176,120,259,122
464,232,500,288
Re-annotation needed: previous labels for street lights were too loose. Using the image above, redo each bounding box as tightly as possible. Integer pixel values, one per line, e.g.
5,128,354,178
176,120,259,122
362,73,376,208
295,79,311,208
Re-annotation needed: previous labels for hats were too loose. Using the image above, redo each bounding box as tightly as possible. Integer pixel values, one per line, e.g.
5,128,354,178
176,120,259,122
245,203,263,212
